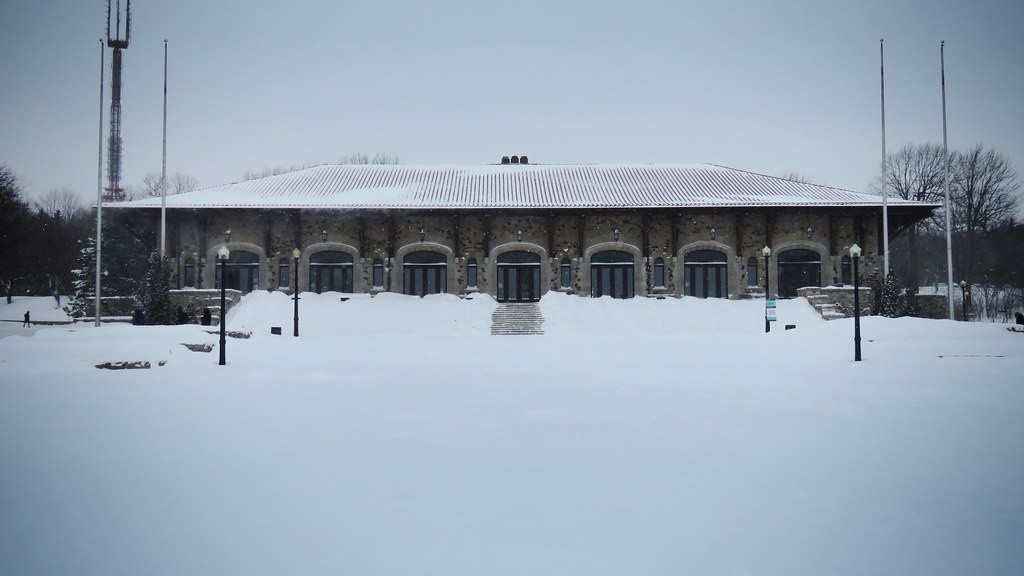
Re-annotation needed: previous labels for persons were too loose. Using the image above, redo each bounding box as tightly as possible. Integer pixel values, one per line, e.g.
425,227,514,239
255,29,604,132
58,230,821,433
5,282,11,303
54,287,60,306
23,311,30,328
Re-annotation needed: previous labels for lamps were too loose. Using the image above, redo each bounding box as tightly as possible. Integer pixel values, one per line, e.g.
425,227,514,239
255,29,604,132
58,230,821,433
321,225,327,243
807,228,813,240
710,228,716,240
517,229,523,241
613,227,619,242
223,224,231,243
419,224,425,242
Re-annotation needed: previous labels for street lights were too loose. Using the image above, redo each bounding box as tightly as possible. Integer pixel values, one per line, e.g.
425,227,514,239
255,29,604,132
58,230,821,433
960,279,966,321
292,248,301,337
217,246,230,365
850,244,861,361
762,246,771,332
